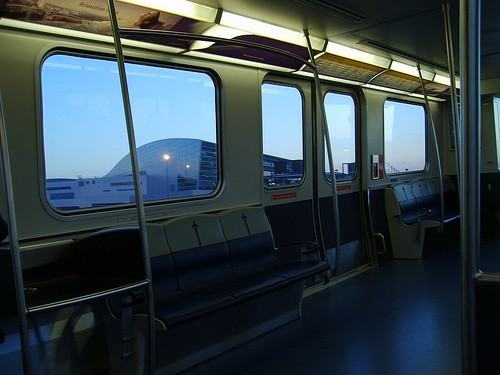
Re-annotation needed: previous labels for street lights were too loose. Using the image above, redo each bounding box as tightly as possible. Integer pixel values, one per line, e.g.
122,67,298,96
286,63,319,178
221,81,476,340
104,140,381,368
184,164,191,177
163,154,170,198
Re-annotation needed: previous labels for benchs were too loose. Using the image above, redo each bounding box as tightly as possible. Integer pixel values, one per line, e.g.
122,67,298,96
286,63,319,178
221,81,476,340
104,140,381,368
71,205,330,375
385,176,461,261
